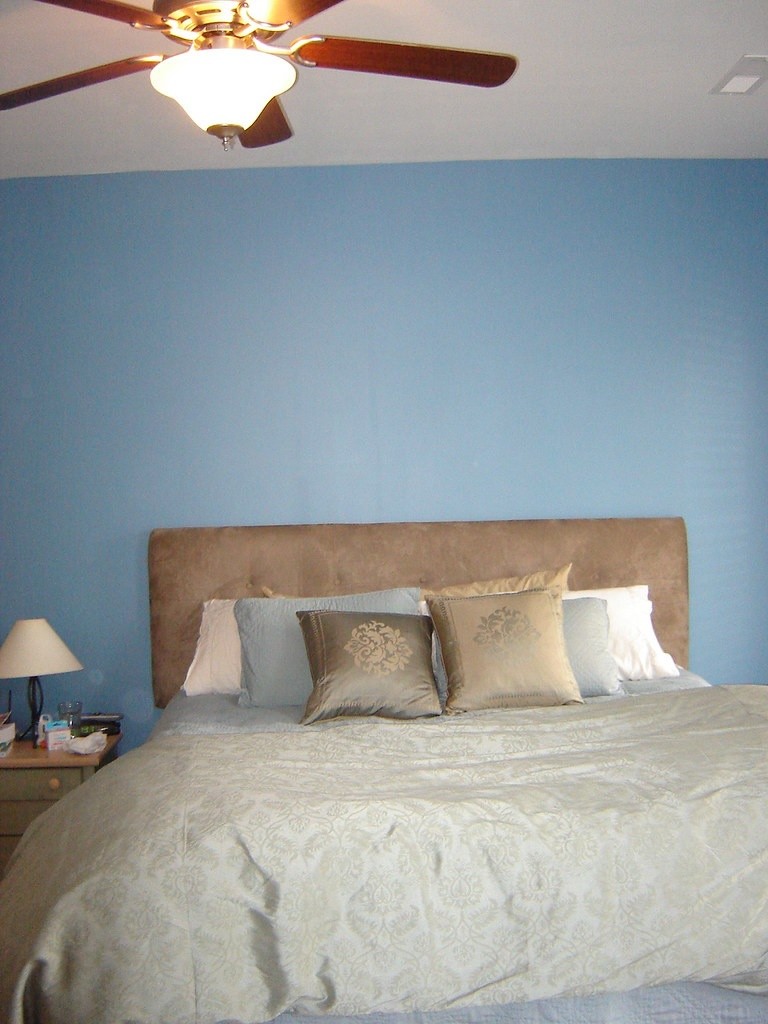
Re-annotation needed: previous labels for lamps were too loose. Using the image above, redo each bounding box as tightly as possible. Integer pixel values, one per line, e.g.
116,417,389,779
0,617,84,739
151,45,297,149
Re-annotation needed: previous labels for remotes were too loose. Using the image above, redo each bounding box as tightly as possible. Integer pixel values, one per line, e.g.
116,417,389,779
82,712,123,722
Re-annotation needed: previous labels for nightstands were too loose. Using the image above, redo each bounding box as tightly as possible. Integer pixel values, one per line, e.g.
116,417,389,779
0,732,122,879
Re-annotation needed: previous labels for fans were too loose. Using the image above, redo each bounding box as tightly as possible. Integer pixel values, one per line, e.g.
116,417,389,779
0,0,514,144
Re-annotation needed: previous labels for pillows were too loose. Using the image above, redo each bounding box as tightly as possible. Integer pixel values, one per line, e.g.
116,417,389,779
293,608,445,727
232,587,431,706
558,596,626,700
425,587,585,715
181,598,247,698
421,560,575,614
558,584,680,685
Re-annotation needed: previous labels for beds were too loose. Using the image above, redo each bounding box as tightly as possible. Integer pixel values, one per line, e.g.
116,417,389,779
2,682,766,1023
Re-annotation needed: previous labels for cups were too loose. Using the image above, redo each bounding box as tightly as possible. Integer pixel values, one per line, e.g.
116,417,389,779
58,701,81,729
